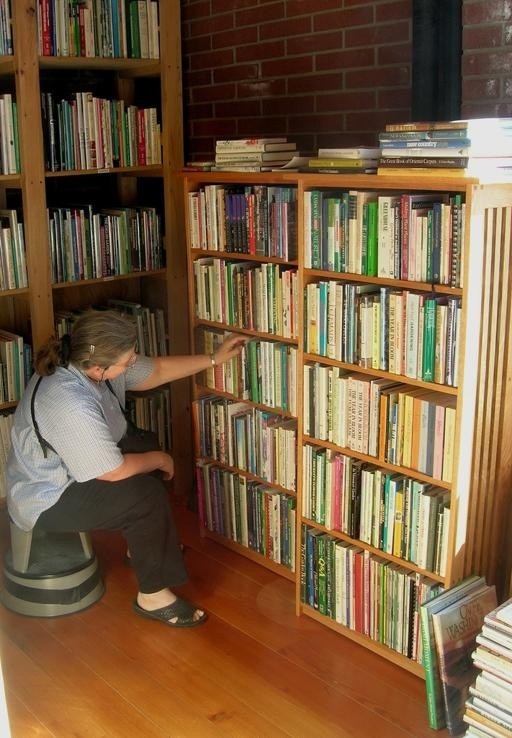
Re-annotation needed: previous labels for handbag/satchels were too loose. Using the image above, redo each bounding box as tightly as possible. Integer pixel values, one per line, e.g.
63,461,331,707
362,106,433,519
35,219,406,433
30,364,160,458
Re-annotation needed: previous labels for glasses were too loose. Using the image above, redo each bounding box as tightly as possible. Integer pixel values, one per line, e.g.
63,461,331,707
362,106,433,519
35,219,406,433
116,351,138,368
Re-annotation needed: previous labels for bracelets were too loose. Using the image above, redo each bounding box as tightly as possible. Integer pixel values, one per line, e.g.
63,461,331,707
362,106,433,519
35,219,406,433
209,352,219,370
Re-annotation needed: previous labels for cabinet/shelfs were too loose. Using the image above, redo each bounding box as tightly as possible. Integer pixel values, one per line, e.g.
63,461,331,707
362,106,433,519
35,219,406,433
0,0,191,493
183,167,484,682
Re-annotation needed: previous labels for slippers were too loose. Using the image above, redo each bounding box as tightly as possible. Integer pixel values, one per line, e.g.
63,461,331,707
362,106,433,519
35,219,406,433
122,545,185,567
132,595,208,627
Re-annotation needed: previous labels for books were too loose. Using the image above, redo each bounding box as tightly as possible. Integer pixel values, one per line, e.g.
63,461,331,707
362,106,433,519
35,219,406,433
457,590,510,737
61,308,162,358
310,142,375,172
310,356,461,483
127,393,180,455
199,387,300,489
305,438,457,583
191,182,299,258
310,186,475,289
40,87,165,171
51,200,171,280
189,257,308,335
421,576,499,733
1,74,23,180
303,523,440,667
2,328,37,407
201,464,298,569
308,281,470,387
1,197,29,296
38,1,154,58
1,5,15,54
214,136,301,173
194,325,300,412
380,114,512,184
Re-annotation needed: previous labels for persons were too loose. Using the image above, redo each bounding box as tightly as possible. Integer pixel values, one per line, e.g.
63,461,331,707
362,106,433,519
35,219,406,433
8,309,250,628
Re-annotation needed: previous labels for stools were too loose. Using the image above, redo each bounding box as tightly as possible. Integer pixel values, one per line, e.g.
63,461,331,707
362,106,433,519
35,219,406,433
6,518,107,618
